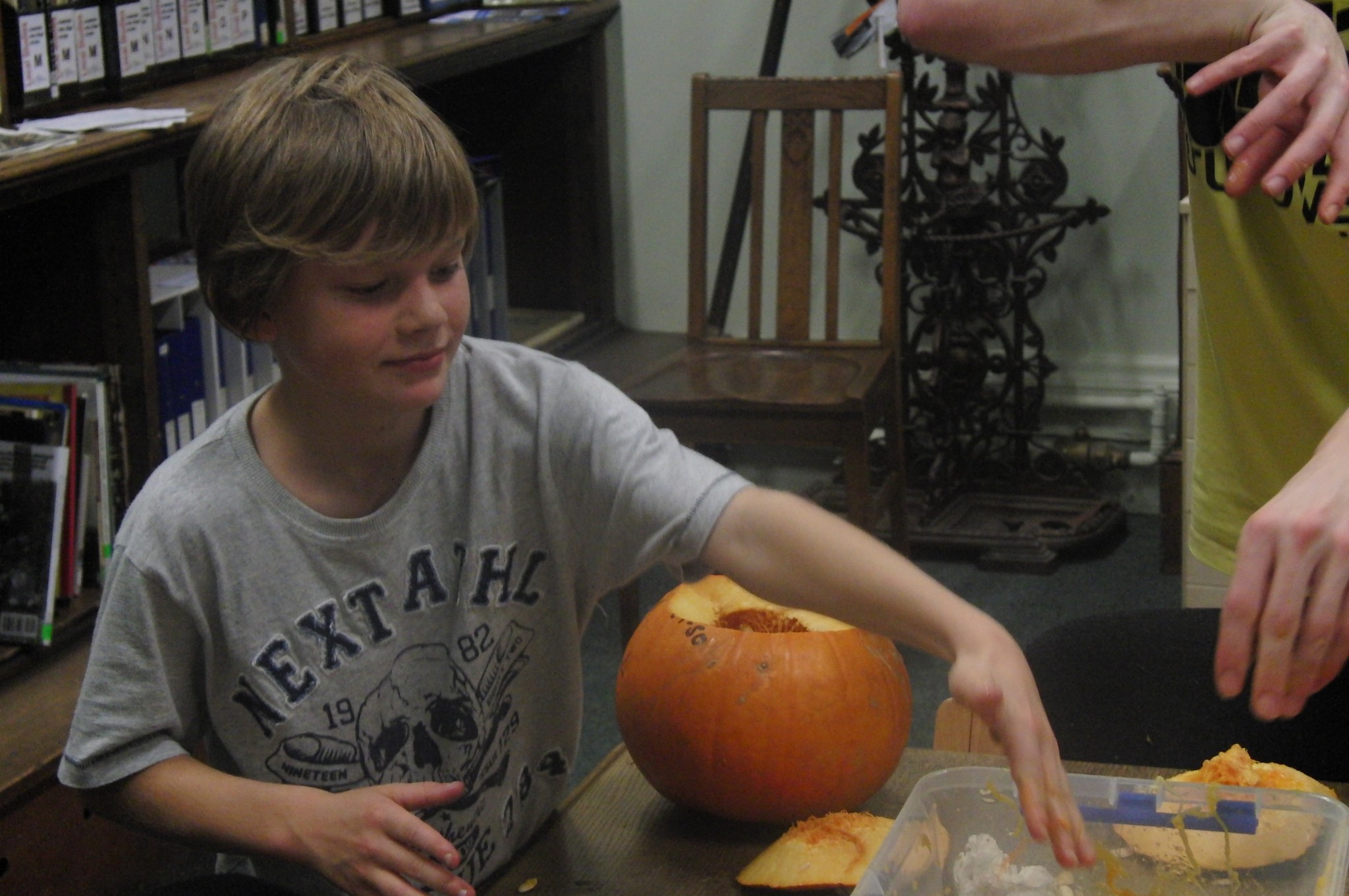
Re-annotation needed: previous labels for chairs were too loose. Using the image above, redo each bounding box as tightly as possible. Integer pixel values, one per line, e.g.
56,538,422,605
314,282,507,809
610,70,914,561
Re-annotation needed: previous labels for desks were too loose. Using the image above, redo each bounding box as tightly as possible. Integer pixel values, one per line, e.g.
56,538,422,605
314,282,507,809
451,728,1347,896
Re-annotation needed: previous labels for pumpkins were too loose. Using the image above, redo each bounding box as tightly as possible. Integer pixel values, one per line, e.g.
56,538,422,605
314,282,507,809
614,572,912,895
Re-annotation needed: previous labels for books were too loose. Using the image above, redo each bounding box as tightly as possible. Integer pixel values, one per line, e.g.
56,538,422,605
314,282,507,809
0,341,151,651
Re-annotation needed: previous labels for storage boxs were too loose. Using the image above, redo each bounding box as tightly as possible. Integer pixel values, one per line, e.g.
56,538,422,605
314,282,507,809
848,763,1347,896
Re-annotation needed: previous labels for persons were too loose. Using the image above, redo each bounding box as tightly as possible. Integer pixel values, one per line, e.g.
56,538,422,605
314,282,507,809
57,49,1095,896
897,1,1349,784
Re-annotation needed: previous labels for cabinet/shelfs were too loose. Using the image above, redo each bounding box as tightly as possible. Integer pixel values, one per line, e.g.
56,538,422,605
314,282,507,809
0,0,617,674
